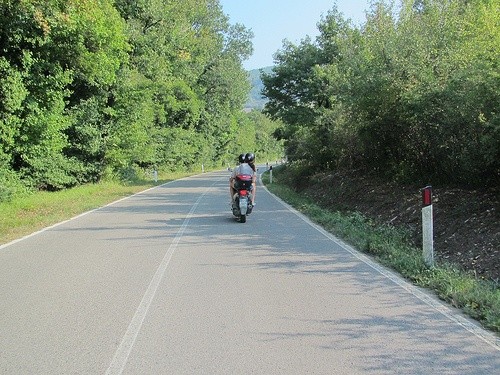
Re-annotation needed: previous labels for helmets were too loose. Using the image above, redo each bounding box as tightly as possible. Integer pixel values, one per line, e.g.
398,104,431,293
239,154,246,163
245,153,255,163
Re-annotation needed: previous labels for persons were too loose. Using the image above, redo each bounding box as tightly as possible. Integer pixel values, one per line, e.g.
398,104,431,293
244,152,257,183
229,153,257,206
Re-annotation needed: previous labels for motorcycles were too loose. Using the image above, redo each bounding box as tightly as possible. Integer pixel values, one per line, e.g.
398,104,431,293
227,168,254,223
